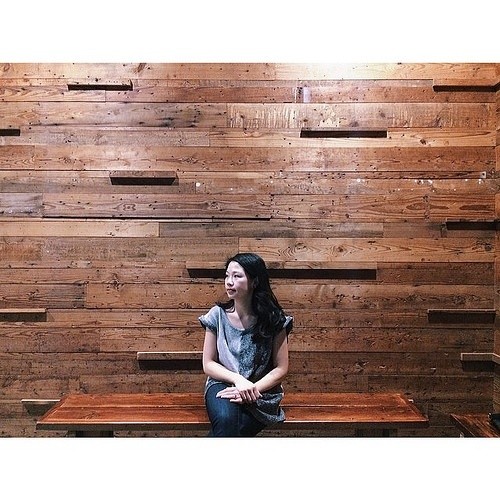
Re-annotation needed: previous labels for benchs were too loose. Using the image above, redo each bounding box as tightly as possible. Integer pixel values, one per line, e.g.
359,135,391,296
34,393,430,437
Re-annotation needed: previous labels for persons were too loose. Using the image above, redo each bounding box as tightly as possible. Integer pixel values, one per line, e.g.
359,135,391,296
199,253,293,438
